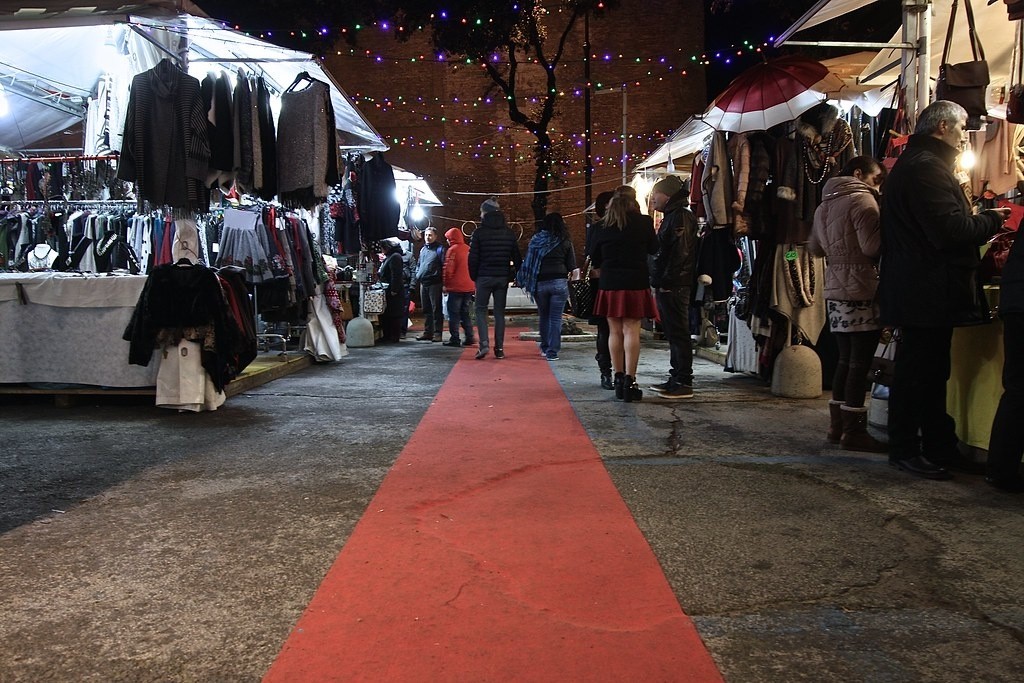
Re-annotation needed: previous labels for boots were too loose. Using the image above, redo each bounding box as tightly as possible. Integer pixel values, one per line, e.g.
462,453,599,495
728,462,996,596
826,399,891,453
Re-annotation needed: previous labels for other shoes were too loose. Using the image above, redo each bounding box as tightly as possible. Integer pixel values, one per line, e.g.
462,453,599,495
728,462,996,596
922,445,984,475
984,471,1024,493
475,347,490,359
540,347,560,361
649,381,693,399
432,336,442,341
443,342,461,347
463,338,477,345
494,347,504,359
416,335,433,341
601,374,615,390
889,453,947,480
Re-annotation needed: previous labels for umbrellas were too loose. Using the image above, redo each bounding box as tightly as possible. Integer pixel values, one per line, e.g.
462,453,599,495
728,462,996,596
701,53,848,134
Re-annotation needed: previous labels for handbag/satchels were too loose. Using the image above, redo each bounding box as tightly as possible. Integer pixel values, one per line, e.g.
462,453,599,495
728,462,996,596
697,305,718,347
440,291,451,321
981,73,1024,125
569,256,604,319
365,288,387,315
1006,85,1024,124
937,60,989,132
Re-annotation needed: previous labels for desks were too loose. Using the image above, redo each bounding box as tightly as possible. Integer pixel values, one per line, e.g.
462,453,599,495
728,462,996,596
0,273,163,388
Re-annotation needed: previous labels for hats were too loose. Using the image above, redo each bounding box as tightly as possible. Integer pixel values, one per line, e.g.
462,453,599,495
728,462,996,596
655,175,680,196
480,196,501,213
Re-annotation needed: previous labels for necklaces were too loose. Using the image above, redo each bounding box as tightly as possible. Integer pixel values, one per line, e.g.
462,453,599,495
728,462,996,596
804,129,835,184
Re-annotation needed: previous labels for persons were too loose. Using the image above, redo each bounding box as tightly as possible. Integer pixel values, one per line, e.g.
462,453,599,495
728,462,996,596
808,156,888,451
468,197,524,359
584,175,740,401
375,226,479,346
518,213,576,359
878,100,1023,491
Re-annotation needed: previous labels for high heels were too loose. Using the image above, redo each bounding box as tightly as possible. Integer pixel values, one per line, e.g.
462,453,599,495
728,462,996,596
612,372,643,402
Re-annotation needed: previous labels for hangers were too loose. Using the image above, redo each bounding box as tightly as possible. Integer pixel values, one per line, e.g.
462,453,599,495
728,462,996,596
0,199,220,225
163,45,265,83
286,62,313,93
225,195,300,217
